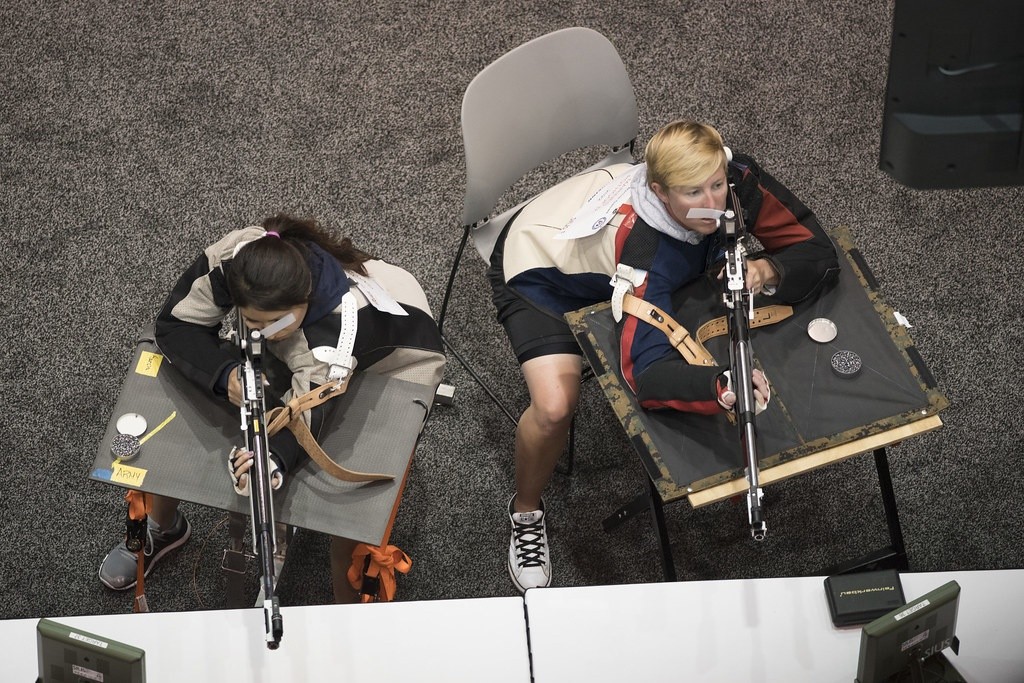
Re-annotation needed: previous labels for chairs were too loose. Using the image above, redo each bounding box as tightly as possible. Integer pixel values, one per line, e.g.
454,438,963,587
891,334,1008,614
439,27,640,486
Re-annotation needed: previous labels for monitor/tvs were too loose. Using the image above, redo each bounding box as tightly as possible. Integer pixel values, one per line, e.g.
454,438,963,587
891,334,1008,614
854,580,962,683
878,0,1024,190
37,618,147,683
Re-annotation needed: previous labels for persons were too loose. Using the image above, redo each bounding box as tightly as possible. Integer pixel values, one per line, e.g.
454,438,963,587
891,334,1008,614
98,215,447,604
487,121,838,593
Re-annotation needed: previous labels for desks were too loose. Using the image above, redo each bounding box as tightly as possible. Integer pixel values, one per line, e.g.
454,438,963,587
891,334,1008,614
89,350,444,604
527,568,1024,683
0,596,529,683
568,265,946,583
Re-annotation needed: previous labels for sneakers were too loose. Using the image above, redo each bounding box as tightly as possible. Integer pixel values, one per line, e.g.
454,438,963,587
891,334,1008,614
99,508,192,590
507,491,552,594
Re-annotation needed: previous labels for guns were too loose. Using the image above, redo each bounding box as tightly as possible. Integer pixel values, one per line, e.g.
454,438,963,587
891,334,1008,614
235,315,285,651
686,184,768,542
